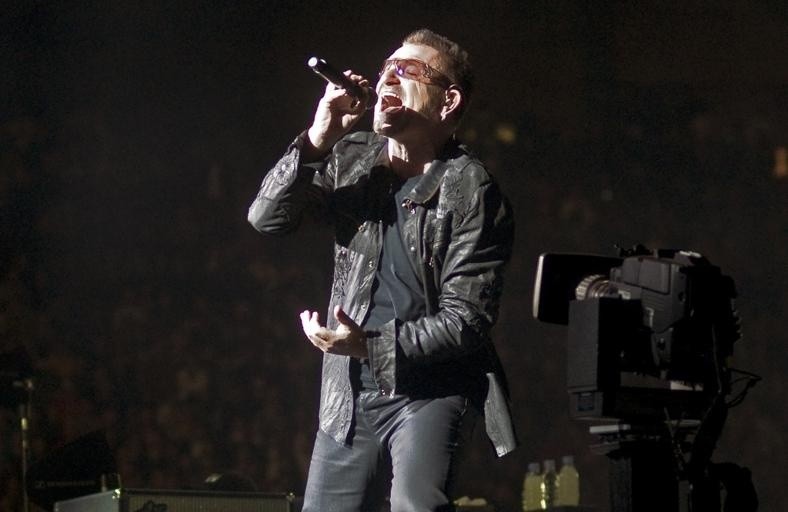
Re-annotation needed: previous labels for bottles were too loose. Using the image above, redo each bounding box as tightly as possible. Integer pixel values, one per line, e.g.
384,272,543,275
556,455,581,508
522,463,547,511
540,458,562,508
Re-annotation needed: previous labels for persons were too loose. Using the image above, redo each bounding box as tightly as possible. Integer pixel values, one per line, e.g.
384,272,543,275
247,25,516,512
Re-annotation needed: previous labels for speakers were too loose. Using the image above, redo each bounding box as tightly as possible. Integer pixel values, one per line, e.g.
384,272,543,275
54,487,295,512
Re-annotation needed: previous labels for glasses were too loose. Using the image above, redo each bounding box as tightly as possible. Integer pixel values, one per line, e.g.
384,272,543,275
378,59,433,82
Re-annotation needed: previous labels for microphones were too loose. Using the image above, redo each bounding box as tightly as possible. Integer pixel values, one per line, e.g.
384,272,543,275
307,56,378,110
